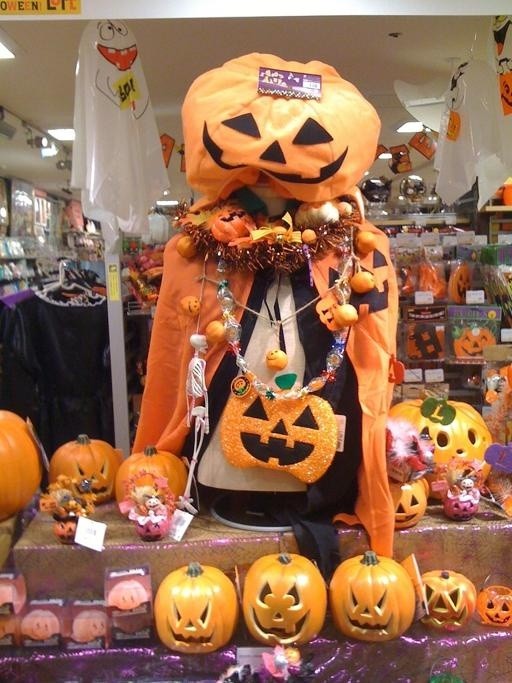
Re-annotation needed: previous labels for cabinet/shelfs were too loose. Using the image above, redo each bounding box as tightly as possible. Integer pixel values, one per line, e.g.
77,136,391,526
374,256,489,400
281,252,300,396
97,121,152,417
0,241,40,303
124,198,512,473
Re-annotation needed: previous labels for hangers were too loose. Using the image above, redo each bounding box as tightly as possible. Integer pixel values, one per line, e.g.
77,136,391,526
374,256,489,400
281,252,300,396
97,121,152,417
43,260,90,299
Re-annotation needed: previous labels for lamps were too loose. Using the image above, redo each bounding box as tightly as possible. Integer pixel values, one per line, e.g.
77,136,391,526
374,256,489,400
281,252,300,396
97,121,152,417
43,145,58,158
33,136,48,144
